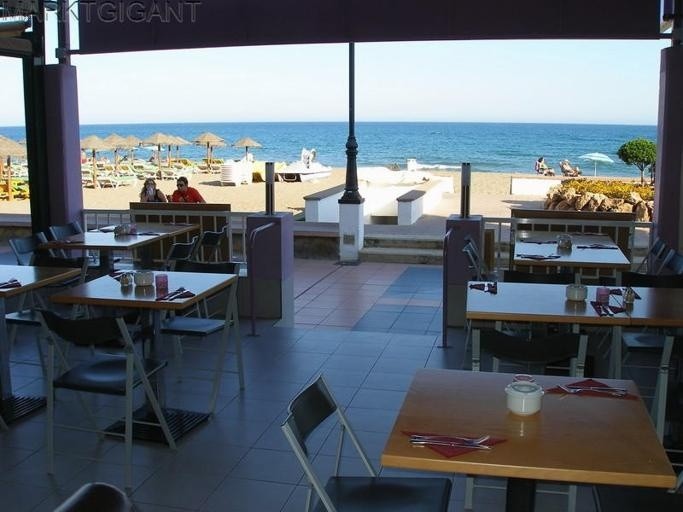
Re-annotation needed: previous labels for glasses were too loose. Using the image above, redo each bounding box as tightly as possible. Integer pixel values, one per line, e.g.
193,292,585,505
176,183,184,188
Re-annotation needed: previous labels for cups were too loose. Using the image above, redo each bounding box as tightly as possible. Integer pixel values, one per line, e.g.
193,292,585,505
130,224,137,235
147,185,153,196
596,287,609,303
504,381,544,418
564,284,587,301
156,274,168,290
556,234,572,248
118,224,131,234
134,272,153,286
511,374,534,383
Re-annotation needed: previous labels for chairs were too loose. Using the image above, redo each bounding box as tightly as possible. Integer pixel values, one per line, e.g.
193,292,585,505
280,375,452,512
81,156,254,188
31,305,181,488
453,235,530,364
559,160,582,177
6,258,90,392
535,160,554,175
156,221,231,270
168,262,245,409
5,221,90,266
593,236,681,376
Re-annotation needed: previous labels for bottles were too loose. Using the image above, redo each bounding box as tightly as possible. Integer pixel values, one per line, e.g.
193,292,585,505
623,283,634,304
114,226,120,234
565,238,571,248
119,272,132,286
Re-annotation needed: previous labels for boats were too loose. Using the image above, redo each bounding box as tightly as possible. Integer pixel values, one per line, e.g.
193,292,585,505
138,142,166,153
275,148,333,184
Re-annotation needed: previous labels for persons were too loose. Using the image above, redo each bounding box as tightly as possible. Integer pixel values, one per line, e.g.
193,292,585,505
140,177,167,203
562,159,580,176
172,177,206,203
537,157,555,176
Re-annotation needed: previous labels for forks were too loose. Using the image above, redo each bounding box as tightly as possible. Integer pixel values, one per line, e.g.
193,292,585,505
410,434,489,444
598,305,606,315
155,287,182,301
554,383,622,398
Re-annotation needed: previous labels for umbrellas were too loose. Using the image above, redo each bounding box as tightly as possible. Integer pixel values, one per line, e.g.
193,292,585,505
80,131,225,188
232,137,261,161
0,130,30,202
578,152,614,176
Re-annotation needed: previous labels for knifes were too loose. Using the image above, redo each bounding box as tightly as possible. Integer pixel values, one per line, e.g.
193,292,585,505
407,441,493,452
483,281,488,293
602,303,614,315
169,289,189,300
0,279,23,289
564,382,629,391
